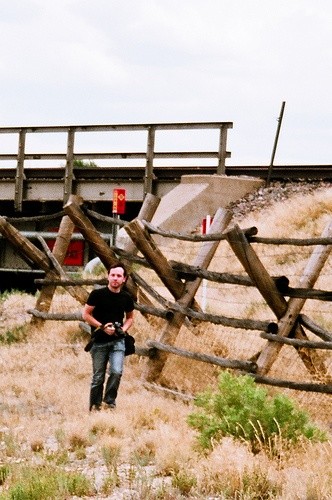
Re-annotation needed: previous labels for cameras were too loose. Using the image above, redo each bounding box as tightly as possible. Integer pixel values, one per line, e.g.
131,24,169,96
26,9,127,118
111,322,122,334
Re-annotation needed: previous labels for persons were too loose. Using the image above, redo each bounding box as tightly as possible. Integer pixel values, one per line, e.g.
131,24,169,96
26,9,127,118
81,261,135,414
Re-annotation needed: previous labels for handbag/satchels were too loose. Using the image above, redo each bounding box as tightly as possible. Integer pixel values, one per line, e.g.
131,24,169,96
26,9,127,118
124,334,136,356
